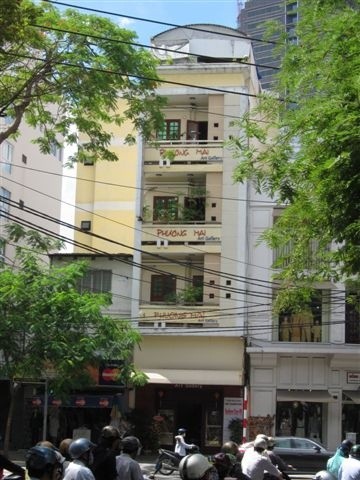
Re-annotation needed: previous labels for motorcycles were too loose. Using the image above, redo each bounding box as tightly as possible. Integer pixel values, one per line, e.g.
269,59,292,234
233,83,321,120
155,443,200,476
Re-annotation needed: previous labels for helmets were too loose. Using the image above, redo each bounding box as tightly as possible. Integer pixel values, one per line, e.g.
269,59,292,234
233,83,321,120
223,441,238,455
25,446,66,477
342,439,354,450
266,437,277,447
178,428,186,432
37,440,59,450
254,439,267,450
69,437,96,459
121,436,143,451
257,434,268,441
60,438,75,457
178,454,212,480
102,425,120,438
350,444,360,455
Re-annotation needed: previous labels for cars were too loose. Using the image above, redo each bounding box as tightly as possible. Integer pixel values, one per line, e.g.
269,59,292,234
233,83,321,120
236,437,336,476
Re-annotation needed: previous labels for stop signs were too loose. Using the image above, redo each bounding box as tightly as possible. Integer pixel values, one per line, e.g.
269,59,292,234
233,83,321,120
102,369,114,381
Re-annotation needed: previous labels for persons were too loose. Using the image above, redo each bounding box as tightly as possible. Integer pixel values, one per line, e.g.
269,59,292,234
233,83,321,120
0,427,360,480
279,303,321,343
